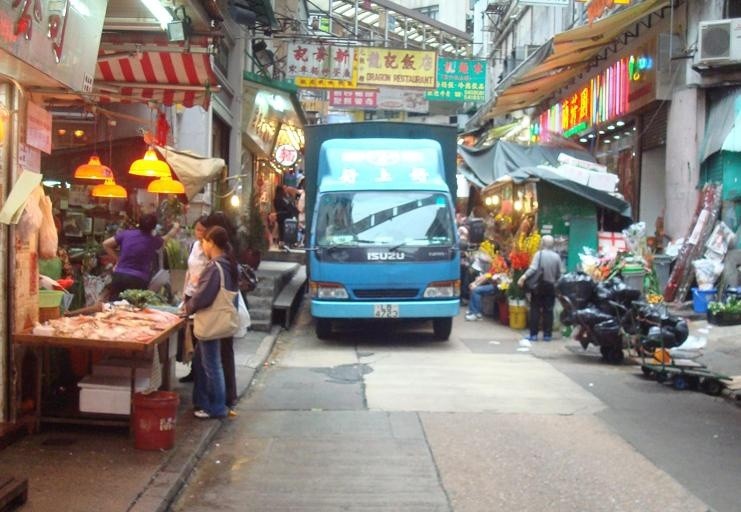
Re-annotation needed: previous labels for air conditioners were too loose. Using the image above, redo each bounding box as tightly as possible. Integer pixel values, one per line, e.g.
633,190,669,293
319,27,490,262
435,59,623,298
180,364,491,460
698,18,741,68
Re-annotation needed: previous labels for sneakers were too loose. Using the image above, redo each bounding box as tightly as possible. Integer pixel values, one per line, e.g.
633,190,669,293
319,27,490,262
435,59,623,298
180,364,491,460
465,311,483,321
525,335,551,341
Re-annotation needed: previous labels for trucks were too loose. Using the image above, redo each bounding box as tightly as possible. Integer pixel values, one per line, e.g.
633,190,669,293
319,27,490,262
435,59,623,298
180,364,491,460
303,120,461,340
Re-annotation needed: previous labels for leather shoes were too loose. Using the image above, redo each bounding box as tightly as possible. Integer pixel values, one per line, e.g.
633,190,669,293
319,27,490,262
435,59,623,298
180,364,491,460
180,373,191,381
194,410,209,418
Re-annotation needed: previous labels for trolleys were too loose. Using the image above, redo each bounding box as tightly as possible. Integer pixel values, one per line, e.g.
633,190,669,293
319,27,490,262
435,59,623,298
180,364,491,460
559,295,733,395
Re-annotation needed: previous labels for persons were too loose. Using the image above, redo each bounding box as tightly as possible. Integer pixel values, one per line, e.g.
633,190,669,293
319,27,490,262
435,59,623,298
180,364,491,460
463,244,511,320
179,211,239,418
517,235,563,340
457,226,471,305
273,174,305,247
102,214,180,301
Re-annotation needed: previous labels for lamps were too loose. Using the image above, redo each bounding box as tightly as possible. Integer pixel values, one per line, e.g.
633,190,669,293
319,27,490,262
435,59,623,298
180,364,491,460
75,99,188,201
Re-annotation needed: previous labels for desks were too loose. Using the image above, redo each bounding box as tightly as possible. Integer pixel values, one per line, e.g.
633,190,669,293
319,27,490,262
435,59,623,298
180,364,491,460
15,304,186,438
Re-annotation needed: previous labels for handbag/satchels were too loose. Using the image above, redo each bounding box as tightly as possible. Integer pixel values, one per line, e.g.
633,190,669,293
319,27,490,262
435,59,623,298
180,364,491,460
193,261,251,341
525,252,544,292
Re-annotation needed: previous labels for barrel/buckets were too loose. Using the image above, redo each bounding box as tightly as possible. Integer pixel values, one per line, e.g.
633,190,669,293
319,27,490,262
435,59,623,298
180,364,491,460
690,287,718,313
132,390,179,451
471,218,484,243
621,268,646,294
510,308,527,329
